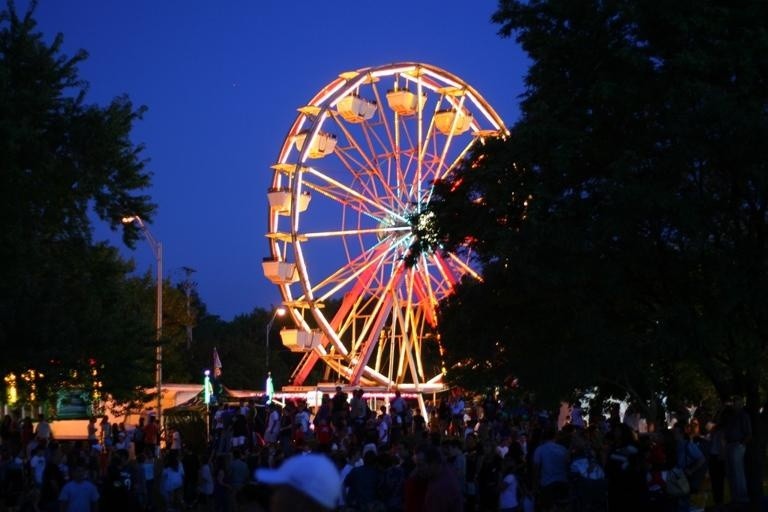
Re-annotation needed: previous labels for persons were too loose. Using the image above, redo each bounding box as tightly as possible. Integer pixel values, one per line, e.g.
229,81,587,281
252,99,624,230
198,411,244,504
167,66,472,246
1,386,767,512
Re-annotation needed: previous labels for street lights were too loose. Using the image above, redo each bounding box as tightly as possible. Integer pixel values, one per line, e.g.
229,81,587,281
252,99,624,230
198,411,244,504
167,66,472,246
265,304,287,373
119,208,164,428
180,265,198,351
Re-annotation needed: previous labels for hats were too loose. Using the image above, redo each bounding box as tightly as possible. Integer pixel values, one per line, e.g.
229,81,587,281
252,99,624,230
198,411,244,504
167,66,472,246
249,451,344,508
